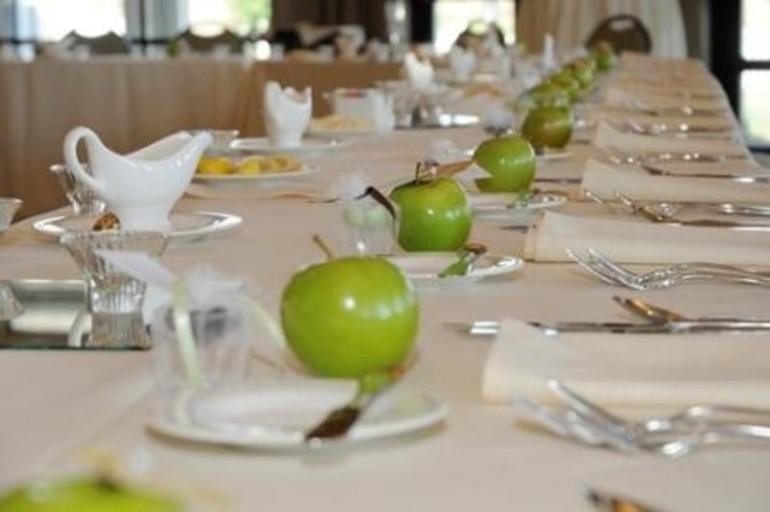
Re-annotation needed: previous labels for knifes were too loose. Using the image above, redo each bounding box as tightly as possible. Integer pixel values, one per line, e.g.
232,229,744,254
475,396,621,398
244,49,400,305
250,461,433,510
301,354,422,440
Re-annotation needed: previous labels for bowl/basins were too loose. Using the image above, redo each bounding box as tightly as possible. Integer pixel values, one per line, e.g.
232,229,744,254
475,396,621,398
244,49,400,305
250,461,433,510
0,197,22,234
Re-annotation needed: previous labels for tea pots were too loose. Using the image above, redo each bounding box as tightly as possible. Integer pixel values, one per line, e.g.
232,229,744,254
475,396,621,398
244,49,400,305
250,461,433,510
63,127,208,231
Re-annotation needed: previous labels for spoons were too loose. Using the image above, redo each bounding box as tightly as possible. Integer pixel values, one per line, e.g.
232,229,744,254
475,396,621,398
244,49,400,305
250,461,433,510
531,110,763,461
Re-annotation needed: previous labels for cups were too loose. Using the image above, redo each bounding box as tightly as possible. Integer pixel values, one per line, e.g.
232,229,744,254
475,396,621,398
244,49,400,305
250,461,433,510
58,233,168,349
346,202,400,253
169,279,253,383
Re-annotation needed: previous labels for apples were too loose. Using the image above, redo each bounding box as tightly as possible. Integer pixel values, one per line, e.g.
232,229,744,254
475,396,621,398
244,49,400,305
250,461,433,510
471,130,536,191
386,161,471,251
514,45,620,154
0,479,186,511
281,233,417,376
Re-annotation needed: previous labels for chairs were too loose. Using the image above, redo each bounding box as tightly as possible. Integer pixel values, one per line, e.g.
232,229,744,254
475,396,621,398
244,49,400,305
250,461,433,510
582,13,650,58
451,21,507,58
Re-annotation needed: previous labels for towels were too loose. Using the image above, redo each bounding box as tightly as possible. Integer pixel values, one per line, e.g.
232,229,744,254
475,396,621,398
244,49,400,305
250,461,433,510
591,119,747,157
520,210,770,270
482,314,769,417
580,157,769,205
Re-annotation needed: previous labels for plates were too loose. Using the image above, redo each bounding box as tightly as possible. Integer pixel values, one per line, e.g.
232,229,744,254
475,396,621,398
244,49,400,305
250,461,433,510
365,114,564,298
36,212,241,247
144,376,445,446
192,79,392,180
